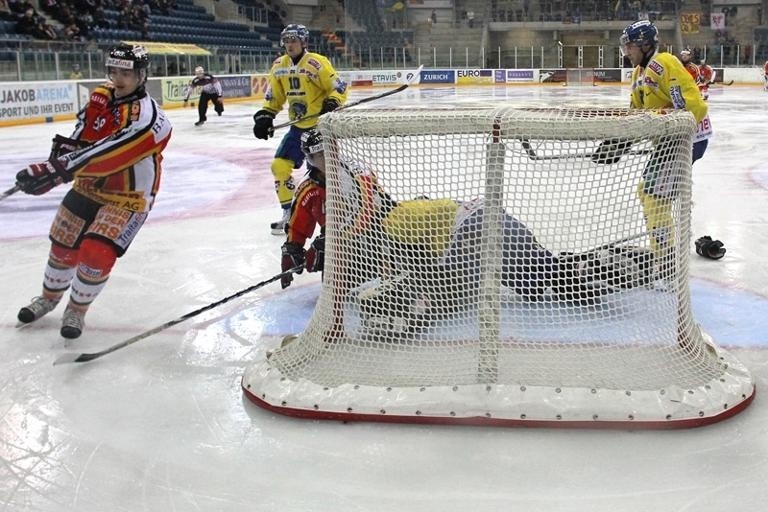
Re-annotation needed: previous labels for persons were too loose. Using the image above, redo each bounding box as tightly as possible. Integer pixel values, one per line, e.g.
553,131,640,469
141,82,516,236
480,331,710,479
349,197,676,343
253,23,350,237
281,129,396,290
230,58,242,75
13,43,173,351
592,20,713,291
678,47,700,87
696,59,713,102
762,60,768,91
0,1,188,80
271,51,282,63
182,66,224,130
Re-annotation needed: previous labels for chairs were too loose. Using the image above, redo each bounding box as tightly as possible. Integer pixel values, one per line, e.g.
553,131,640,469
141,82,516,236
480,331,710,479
0,1,416,84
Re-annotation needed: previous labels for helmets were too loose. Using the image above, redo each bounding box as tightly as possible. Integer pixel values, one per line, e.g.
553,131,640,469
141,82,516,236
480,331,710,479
619,19,659,49
105,42,151,70
300,129,333,155
194,67,204,74
280,23,309,47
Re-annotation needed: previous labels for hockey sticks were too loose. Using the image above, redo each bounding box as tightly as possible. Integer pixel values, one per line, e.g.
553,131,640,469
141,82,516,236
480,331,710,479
714,80,733,86
268,64,423,131
54,265,306,364
518,138,653,161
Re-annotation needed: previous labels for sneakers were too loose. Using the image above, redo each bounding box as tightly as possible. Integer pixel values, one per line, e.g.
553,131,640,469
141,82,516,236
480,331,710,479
271,208,291,229
61,304,85,339
19,296,59,323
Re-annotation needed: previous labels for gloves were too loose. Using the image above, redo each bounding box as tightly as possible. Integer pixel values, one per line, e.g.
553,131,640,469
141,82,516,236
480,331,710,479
319,98,339,114
696,236,726,259
304,235,324,272
16,158,74,195
282,236,306,288
254,110,275,140
